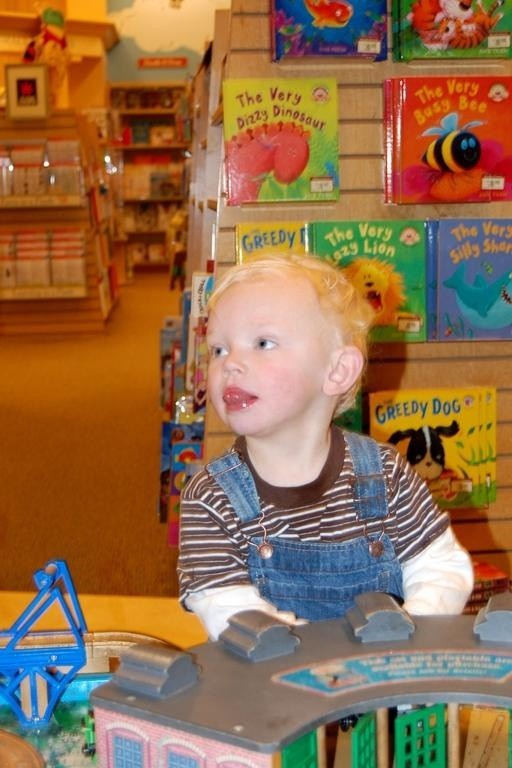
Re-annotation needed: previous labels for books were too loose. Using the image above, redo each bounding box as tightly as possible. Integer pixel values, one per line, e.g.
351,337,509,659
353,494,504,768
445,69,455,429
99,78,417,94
0,108,123,319
113,88,183,265
162,1,512,511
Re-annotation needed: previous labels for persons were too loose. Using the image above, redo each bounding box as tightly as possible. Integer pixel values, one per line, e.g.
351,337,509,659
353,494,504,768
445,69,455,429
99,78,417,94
175,245,475,640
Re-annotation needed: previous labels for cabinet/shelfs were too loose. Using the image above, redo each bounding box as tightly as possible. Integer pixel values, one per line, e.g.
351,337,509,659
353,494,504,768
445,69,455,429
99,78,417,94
0,1,123,336
109,80,187,272
177,1,512,556
